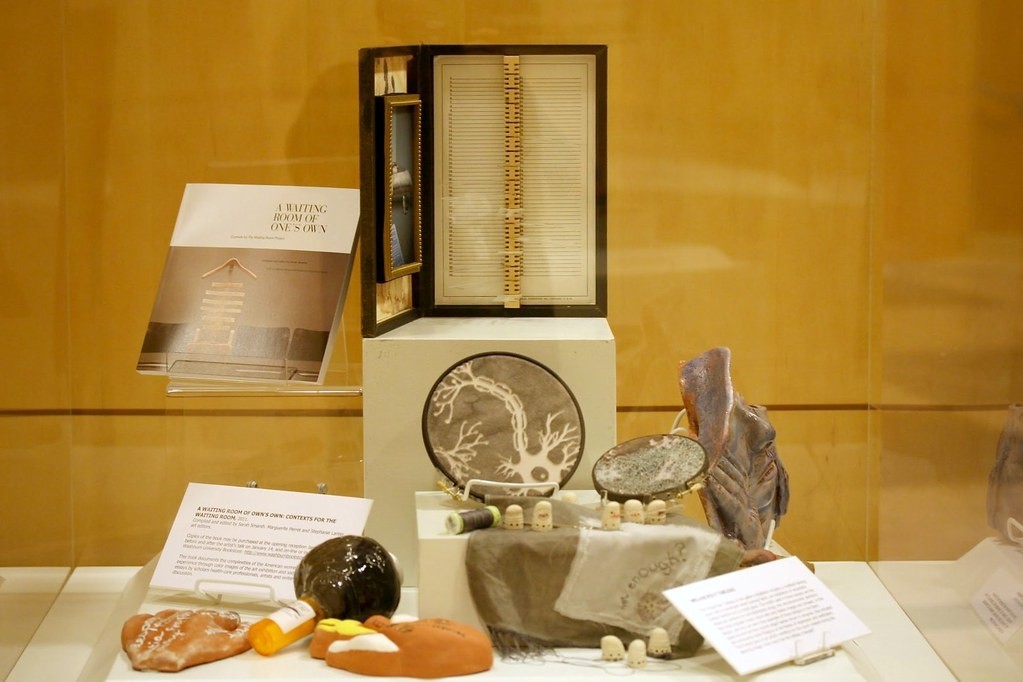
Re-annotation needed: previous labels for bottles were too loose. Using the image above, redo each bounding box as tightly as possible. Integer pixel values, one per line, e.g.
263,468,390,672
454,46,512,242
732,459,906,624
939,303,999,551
246,535,401,657
986,401,1023,548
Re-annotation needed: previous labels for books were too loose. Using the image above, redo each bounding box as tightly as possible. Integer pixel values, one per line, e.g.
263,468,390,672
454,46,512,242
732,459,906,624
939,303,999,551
134,183,361,385
